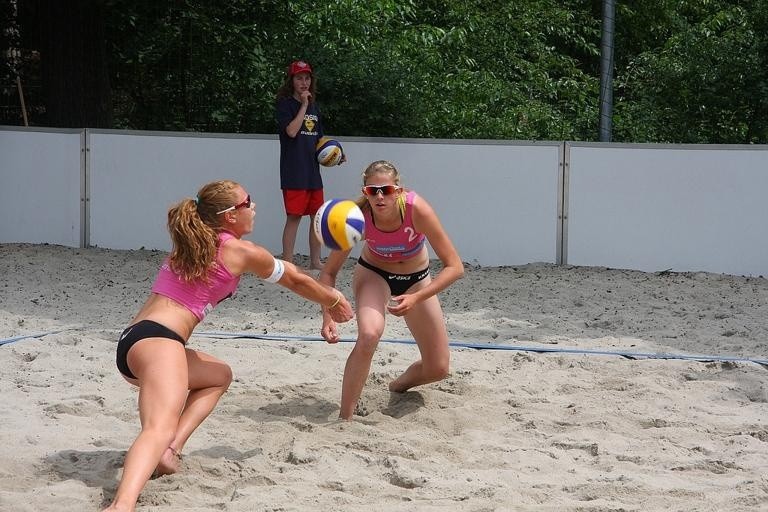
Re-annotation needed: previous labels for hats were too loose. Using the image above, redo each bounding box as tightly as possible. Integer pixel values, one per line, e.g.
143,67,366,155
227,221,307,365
286,60,313,77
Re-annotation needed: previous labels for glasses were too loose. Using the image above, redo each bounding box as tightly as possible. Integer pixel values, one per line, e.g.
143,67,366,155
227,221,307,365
360,183,402,196
215,194,252,214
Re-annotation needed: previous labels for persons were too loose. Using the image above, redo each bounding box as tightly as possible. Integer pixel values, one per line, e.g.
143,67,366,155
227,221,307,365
271,59,349,277
97,180,358,512
318,160,465,424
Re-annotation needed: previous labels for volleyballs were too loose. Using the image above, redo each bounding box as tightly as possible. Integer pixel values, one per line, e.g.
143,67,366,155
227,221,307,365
316,138,342,167
314,198,365,252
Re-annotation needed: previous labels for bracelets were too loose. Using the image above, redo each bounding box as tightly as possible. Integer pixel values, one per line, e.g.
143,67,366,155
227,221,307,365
328,294,341,313
